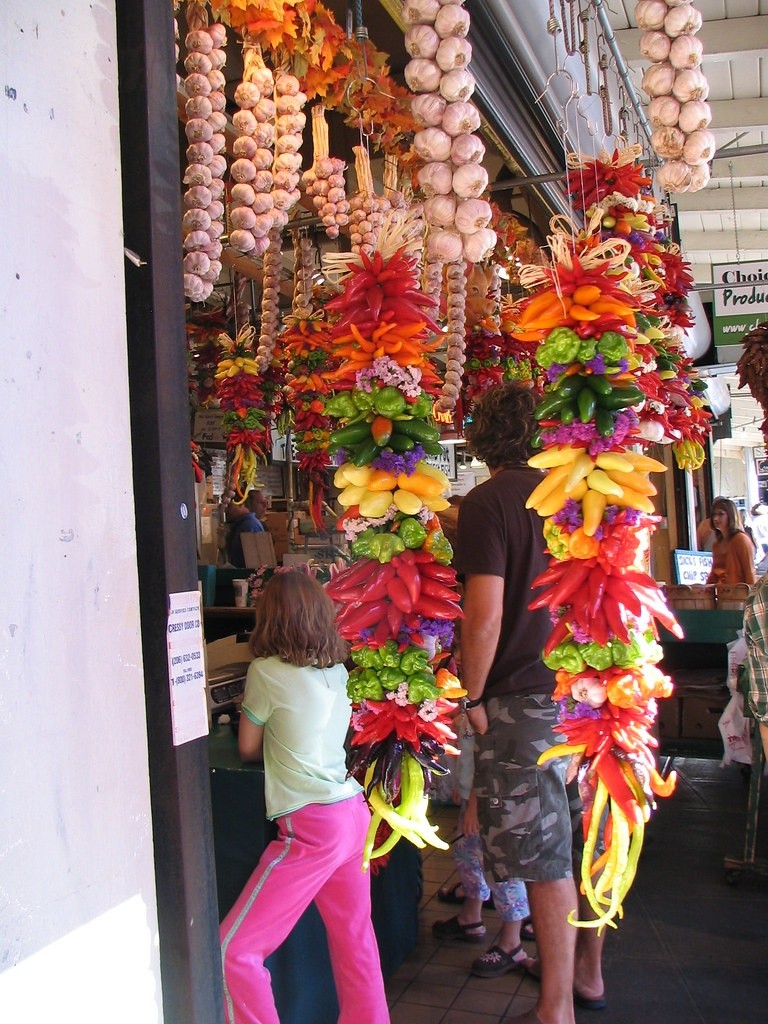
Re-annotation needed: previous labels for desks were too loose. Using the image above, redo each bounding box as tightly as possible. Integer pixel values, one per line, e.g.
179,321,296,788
209,723,419,1024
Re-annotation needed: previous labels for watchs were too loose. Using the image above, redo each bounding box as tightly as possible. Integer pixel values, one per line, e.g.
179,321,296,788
461,696,482,710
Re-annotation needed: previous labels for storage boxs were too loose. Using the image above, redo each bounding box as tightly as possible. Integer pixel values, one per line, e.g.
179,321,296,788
266,512,306,563
657,690,731,739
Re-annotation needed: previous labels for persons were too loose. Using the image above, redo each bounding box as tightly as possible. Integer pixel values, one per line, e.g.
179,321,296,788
722,571,768,886
697,496,768,584
218,571,391,1024
433,383,608,1023
227,490,267,568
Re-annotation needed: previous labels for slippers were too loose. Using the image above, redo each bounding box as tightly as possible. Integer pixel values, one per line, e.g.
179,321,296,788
524,957,607,1010
504,1009,541,1024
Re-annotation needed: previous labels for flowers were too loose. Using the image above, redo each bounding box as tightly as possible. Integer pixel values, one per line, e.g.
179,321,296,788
541,409,628,455
554,496,643,541
350,681,438,731
548,604,650,645
557,694,602,725
343,504,435,543
355,355,422,397
360,615,455,647
334,443,425,479
545,355,628,380
247,564,306,608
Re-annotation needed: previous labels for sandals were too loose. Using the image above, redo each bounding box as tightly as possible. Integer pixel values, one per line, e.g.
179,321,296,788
438,881,495,910
471,943,528,977
522,921,536,940
431,915,486,943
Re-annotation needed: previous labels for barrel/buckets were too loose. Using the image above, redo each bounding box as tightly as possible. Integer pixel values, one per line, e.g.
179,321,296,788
666,584,716,611
716,581,753,612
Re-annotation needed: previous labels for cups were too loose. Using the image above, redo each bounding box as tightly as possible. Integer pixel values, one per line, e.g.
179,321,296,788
232,578,248,607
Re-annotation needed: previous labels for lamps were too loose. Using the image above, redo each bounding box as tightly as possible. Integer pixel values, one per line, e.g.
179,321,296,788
459,452,466,470
440,424,466,445
